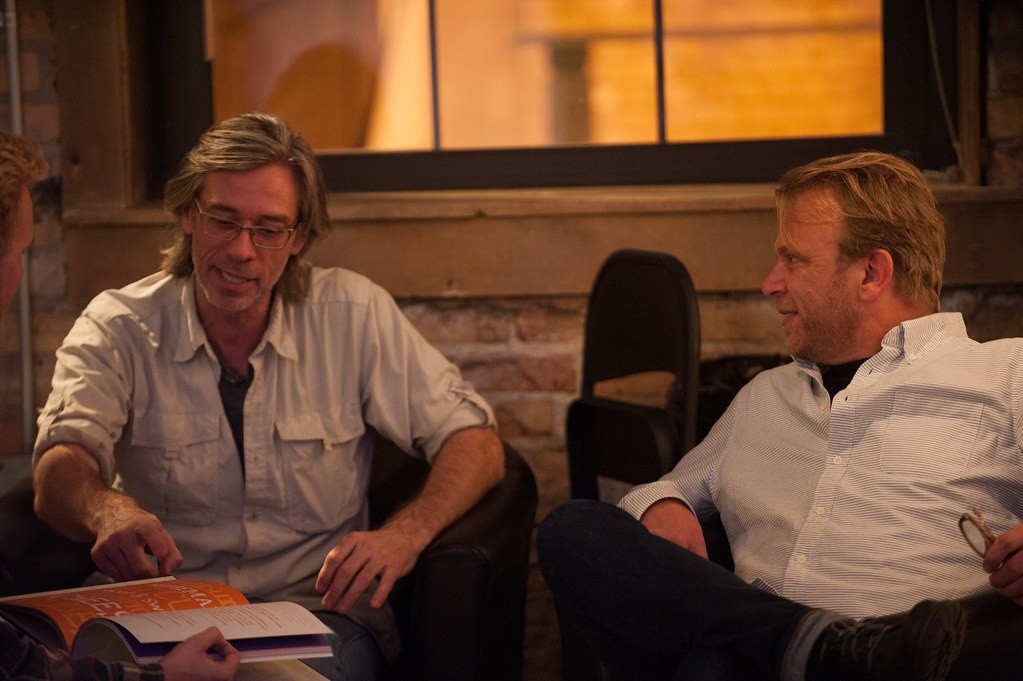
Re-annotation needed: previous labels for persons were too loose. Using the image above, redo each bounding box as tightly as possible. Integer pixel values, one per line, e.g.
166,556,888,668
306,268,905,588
0,133,240,681
534,153,1023,681
32,112,505,681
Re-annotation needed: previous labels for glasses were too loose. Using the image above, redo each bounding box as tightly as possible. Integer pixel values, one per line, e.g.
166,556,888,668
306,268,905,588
194,197,296,250
959,505,995,556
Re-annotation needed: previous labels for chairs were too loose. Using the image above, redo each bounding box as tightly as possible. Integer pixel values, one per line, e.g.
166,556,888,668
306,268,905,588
0,436,537,681
567,246,718,501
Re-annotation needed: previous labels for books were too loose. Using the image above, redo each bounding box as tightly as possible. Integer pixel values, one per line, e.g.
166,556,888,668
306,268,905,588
0,576,334,667
